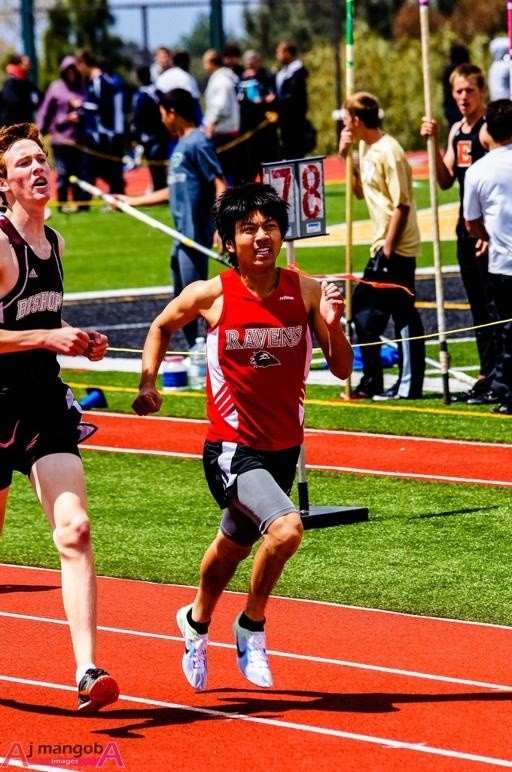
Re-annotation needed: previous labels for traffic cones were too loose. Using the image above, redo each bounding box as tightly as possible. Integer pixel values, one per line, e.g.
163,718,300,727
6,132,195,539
81,387,108,412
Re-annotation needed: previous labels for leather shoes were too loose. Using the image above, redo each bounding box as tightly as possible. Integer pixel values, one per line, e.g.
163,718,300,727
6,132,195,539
452,389,510,414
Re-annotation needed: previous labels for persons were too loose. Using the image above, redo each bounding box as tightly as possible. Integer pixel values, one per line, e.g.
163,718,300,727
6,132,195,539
0,41,317,221
463,98,512,415
0,123,119,713
159,87,228,349
131,183,353,692
443,45,469,127
487,36,512,102
421,62,493,405
339,90,425,402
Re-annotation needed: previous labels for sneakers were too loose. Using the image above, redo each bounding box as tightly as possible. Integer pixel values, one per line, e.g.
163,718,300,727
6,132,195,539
174,602,208,692
335,383,404,401
75,668,120,719
234,615,278,690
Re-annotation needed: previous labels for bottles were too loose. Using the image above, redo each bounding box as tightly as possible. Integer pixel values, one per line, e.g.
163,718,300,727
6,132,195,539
189,335,208,389
162,355,188,392
